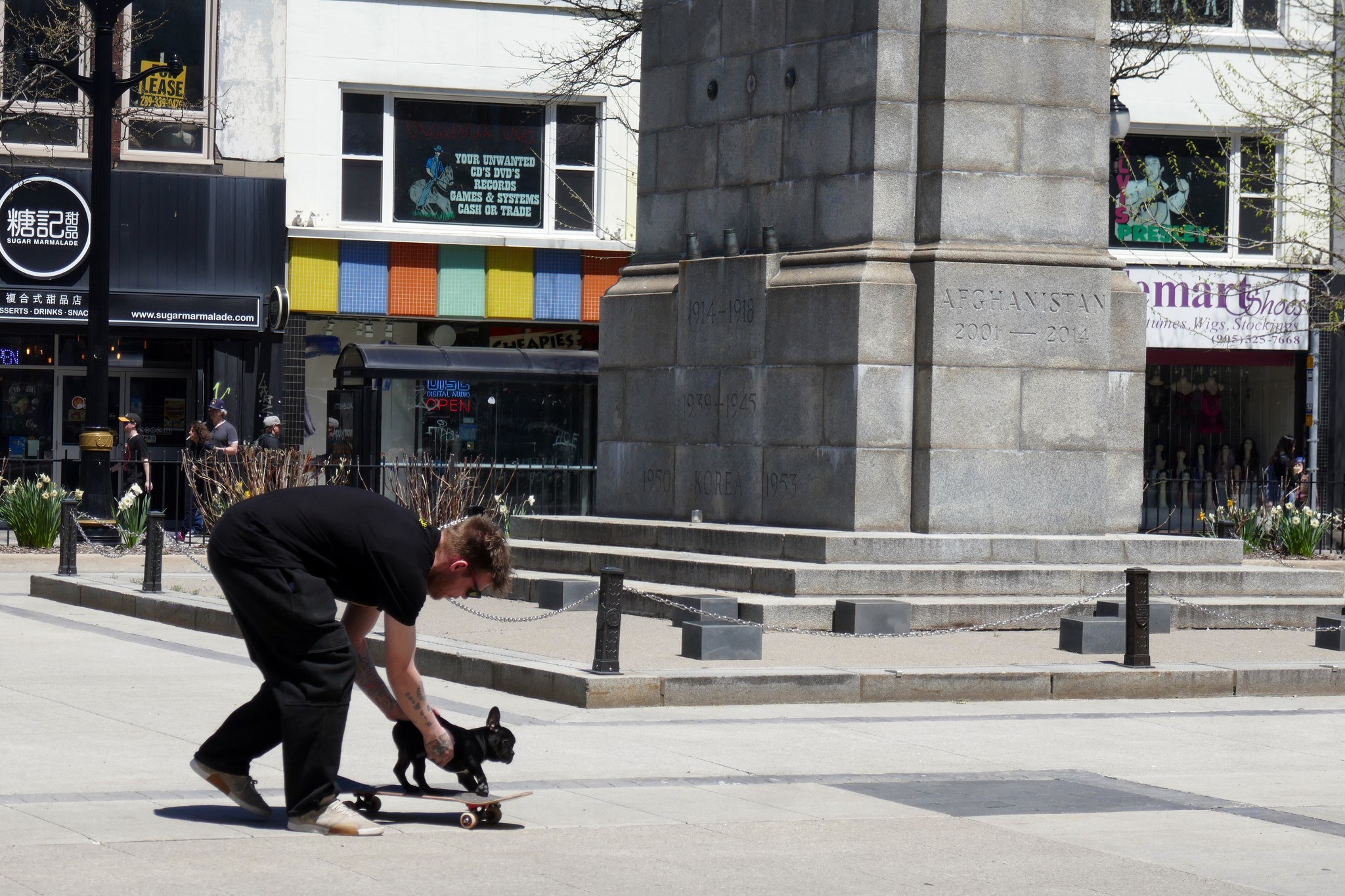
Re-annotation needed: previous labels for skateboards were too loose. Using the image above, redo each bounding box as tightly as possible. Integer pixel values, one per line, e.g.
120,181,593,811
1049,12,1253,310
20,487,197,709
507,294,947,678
332,774,534,830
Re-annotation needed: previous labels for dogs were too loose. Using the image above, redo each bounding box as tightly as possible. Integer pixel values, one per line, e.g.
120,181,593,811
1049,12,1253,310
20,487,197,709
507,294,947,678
392,706,515,797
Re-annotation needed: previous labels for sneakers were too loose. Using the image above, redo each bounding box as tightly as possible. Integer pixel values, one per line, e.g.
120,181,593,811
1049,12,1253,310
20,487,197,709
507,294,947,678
189,757,273,817
287,798,384,836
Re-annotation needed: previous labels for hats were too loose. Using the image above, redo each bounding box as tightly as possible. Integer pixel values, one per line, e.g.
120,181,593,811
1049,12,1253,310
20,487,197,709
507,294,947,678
263,416,286,428
118,413,141,426
207,399,225,409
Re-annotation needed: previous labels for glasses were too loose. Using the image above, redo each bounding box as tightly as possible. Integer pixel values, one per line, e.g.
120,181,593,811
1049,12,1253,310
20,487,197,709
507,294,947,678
461,558,481,599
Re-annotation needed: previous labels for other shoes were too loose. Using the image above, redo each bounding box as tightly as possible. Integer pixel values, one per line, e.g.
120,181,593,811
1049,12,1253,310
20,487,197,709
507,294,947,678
171,532,185,543
185,530,200,537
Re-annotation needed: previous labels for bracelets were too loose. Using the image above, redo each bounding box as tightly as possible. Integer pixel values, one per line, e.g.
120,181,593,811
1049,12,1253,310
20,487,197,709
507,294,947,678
110,468,112,474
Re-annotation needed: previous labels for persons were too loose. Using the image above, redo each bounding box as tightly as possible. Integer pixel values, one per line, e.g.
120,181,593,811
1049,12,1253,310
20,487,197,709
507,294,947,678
191,485,515,837
169,399,238,543
109,413,153,530
1145,376,1260,508
256,416,286,492
1264,435,1320,548
328,418,353,485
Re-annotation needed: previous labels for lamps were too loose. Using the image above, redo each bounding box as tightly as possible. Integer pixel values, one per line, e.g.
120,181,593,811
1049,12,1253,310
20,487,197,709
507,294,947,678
762,225,779,254
295,210,316,226
1110,82,1132,145
606,224,625,240
1173,365,1249,377
365,318,374,338
685,232,702,260
353,319,364,336
723,228,739,257
325,317,334,336
385,319,393,337
